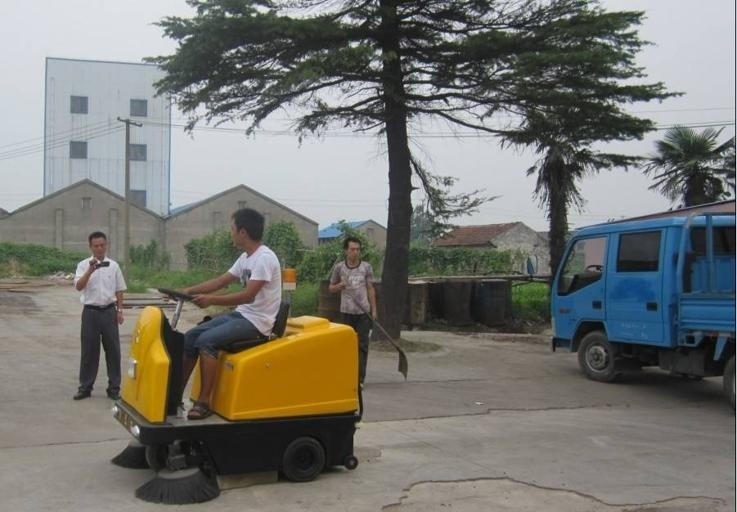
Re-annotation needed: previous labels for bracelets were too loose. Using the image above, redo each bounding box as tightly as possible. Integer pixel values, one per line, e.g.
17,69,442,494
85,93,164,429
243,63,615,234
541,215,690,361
117,309,123,312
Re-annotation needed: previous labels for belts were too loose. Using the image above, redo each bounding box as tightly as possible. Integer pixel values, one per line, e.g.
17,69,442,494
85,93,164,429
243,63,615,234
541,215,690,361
85,302,116,311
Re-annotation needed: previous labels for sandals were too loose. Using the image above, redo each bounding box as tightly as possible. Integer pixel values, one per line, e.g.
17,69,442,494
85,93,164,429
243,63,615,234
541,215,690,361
188,400,210,420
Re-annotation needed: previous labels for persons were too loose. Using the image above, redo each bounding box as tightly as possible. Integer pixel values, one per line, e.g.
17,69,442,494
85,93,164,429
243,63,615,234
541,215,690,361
328,237,377,390
177,207,282,420
71,232,128,401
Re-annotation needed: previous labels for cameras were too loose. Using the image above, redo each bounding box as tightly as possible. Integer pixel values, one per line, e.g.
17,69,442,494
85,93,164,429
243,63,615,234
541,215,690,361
94,260,110,268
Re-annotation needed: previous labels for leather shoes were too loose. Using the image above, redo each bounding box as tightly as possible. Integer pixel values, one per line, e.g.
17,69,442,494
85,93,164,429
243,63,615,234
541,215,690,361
73,392,91,400
107,388,120,401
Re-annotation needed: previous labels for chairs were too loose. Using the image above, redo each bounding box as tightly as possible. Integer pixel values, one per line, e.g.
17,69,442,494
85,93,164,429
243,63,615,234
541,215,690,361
219,299,290,353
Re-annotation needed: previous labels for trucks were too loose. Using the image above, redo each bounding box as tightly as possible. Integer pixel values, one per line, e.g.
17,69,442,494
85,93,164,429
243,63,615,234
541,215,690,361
549,213,736,410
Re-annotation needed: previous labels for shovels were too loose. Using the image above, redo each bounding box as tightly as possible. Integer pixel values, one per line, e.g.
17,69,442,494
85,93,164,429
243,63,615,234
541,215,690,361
344,285,408,380
